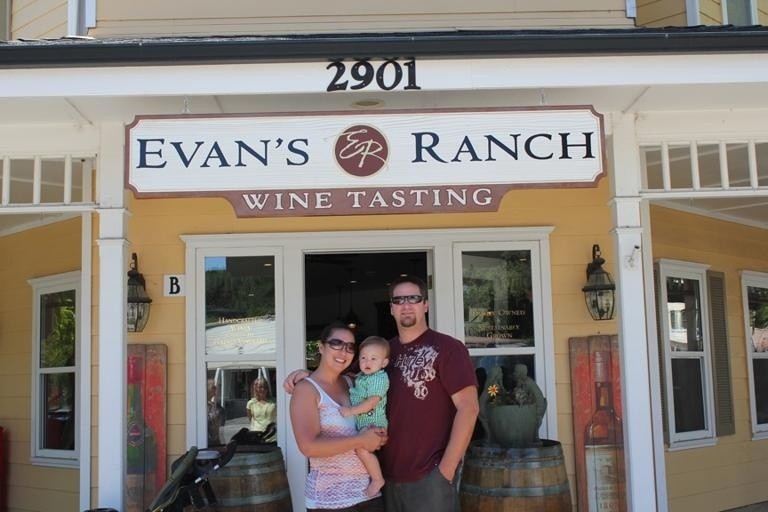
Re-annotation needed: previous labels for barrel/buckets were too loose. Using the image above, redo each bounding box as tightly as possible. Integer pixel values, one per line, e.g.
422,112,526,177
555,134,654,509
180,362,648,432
455,439,573,512
195,443,293,512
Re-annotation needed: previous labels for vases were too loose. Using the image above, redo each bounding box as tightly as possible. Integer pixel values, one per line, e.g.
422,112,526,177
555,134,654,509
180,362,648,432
478,364,548,445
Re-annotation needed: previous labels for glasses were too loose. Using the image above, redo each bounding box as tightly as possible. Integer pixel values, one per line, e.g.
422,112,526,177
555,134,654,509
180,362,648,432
392,295,427,305
324,339,356,353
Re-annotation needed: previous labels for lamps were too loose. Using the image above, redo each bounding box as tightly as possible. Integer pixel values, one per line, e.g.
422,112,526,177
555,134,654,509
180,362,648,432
578,244,618,323
126,251,152,334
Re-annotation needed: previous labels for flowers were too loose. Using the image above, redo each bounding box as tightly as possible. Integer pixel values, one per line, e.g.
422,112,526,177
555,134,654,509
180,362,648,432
488,382,504,402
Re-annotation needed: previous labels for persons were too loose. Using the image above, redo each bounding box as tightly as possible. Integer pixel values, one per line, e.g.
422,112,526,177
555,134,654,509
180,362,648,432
283,276,480,512
513,364,546,442
246,378,276,442
479,365,504,444
290,323,389,512
207,379,221,445
339,336,391,497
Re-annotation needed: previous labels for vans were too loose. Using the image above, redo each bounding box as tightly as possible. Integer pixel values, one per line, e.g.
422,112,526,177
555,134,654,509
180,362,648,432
668,302,703,351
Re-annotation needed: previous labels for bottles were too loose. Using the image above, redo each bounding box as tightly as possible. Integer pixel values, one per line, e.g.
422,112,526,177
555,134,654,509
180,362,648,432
583,350,627,511
123,355,157,511
